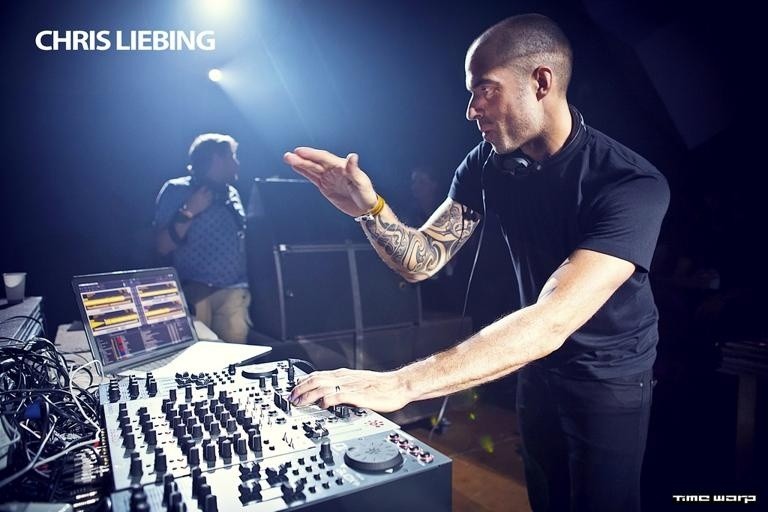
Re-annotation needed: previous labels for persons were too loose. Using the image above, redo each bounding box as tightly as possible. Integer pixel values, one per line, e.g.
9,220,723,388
279,12,676,510
148,130,256,346
398,161,448,229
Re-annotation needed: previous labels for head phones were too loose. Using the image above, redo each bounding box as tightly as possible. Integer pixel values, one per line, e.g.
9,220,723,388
481,104,584,176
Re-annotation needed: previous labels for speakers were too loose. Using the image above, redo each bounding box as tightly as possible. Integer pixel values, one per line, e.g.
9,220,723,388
350,243,422,331
248,243,358,339
244,177,369,244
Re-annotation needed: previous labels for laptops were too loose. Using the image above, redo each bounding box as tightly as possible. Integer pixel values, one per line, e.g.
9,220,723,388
71,266,273,380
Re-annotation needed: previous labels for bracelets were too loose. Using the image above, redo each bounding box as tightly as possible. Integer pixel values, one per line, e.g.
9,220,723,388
165,207,195,247
350,192,386,225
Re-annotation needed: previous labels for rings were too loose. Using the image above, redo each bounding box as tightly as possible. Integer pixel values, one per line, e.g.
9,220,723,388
334,384,343,394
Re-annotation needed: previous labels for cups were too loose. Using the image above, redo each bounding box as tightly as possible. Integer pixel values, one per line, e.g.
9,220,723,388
2,271,27,305
